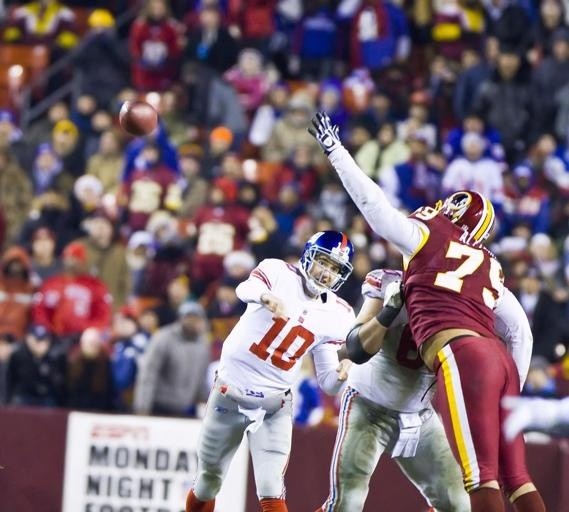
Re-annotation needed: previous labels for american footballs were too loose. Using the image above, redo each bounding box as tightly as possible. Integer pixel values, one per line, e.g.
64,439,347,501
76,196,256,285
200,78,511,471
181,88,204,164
119,98,159,136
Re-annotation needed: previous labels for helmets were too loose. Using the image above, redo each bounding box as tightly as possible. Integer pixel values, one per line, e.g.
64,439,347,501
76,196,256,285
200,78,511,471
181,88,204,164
435,190,495,243
298,230,355,295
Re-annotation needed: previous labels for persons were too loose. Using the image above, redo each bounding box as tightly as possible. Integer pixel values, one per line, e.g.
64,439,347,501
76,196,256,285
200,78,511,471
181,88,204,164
3,4,568,440
305,110,549,512
186,230,357,512
310,268,473,512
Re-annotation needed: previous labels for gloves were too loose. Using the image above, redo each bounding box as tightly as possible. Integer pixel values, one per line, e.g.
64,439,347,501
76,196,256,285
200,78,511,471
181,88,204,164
307,112,341,158
383,280,406,310
